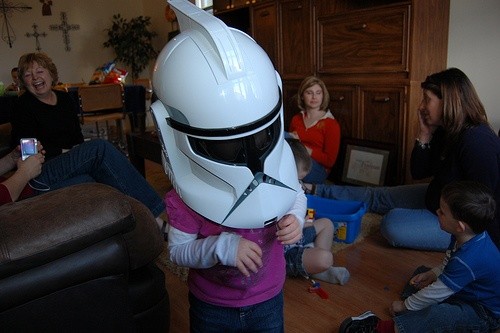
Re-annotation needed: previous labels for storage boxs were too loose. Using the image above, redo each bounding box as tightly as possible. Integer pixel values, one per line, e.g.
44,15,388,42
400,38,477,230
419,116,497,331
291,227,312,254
304,195,369,244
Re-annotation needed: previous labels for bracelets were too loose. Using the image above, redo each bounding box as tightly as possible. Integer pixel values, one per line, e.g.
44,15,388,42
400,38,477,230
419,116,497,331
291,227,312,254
415,137,432,147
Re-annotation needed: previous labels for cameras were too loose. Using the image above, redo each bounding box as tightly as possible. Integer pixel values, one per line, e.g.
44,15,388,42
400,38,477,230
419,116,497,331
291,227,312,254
20,138,38,161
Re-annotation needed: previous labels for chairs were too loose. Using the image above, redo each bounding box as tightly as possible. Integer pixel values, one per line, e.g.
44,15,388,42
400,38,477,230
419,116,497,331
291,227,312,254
79,84,126,140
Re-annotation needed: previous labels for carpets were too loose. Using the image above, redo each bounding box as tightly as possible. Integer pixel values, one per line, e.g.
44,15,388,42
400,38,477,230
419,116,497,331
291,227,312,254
156,212,384,283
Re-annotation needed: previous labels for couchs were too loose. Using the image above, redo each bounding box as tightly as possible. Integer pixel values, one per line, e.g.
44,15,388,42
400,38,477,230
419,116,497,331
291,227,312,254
0,182,173,333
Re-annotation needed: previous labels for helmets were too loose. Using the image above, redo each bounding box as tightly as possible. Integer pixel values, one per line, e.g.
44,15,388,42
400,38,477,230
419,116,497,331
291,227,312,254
149,0,298,229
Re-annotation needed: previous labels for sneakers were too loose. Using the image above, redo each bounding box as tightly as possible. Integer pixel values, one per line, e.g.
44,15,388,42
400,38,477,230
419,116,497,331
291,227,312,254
338,311,381,333
159,220,169,243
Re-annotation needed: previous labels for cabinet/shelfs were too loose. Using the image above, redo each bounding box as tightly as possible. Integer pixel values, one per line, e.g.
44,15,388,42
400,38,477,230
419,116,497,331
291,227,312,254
213,0,450,185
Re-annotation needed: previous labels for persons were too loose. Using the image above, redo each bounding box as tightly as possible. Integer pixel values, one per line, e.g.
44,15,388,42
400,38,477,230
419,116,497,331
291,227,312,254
11,53,84,160
299,67,500,252
283,138,351,285
338,181,500,333
4,67,22,96
165,104,307,333
0,139,169,242
284,77,340,184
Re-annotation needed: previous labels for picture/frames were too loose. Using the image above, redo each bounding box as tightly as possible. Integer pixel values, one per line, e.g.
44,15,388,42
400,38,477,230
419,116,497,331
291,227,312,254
336,137,399,188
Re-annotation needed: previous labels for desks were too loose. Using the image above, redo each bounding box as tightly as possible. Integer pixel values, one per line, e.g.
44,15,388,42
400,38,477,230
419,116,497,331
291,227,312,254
0,84,147,180
